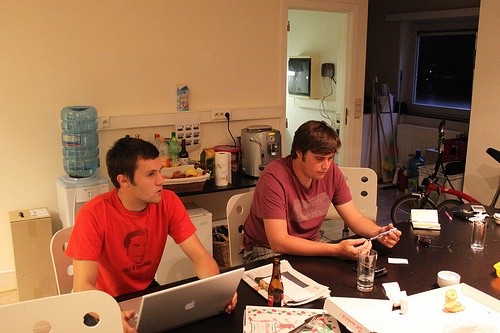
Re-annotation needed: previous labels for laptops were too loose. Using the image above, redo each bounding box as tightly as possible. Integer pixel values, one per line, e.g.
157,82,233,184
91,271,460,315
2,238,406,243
118,266,245,333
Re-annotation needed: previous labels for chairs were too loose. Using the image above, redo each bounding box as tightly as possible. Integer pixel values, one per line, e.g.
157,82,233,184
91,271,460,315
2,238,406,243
324,167,378,231
51,226,78,295
226,190,259,267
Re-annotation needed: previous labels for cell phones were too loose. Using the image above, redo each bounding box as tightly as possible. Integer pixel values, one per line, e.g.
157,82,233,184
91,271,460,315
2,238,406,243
359,259,386,273
471,204,487,213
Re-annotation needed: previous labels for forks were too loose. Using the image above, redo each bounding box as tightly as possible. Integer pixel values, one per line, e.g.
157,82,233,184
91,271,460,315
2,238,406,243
295,317,318,333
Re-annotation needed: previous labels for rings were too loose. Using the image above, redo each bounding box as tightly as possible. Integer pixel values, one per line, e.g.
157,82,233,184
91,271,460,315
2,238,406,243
394,237,397,239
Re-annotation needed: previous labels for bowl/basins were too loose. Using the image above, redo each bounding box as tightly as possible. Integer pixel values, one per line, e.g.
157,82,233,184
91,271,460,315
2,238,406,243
437,271,461,288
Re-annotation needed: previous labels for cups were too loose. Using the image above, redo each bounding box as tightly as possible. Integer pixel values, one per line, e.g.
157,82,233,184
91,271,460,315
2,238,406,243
471,220,487,251
357,249,377,292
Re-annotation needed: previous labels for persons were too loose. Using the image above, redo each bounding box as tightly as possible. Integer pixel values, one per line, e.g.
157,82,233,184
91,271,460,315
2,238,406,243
66,134,238,333
240,120,402,264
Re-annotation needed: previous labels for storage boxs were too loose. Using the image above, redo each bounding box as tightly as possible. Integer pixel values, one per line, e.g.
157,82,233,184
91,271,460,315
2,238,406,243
417,161,465,208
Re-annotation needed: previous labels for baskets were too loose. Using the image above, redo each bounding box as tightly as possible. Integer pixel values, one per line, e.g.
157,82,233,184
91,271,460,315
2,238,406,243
163,181,205,193
213,233,229,266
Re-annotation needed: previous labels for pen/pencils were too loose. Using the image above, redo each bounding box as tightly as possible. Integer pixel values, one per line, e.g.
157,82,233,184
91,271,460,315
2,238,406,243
365,227,397,243
444,211,453,220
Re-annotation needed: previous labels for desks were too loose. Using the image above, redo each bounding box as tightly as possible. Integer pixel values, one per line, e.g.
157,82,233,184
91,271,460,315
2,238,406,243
8,207,54,300
106,203,500,333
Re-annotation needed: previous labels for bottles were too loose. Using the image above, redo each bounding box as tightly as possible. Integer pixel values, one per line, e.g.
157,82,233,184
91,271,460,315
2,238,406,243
152,133,166,169
164,132,180,162
179,139,189,166
407,149,425,191
342,224,350,238
268,258,284,307
61,106,99,178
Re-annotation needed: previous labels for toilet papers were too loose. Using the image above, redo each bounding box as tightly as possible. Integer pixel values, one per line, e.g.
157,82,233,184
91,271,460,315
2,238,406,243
379,95,393,112
381,81,387,95
215,152,231,186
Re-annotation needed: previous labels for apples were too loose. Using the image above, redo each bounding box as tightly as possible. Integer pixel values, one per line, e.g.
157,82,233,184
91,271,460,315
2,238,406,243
162,165,203,179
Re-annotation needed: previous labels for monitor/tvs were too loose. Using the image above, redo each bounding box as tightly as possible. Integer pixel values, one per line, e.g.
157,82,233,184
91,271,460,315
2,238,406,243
288,57,310,96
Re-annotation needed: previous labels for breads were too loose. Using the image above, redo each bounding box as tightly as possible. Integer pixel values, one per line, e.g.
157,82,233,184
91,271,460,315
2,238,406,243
444,301,465,312
444,290,457,302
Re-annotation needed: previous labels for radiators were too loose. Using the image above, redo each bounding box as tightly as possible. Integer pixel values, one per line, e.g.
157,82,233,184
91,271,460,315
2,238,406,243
396,124,463,182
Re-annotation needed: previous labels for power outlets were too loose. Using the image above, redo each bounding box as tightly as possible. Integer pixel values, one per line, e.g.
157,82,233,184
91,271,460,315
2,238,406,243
99,118,111,130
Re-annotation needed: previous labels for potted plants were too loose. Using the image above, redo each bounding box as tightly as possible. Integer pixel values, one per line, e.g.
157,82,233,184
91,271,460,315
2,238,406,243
212,111,231,120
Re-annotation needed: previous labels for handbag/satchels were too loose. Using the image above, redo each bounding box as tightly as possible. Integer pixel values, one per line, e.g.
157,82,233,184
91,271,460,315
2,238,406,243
214,225,228,242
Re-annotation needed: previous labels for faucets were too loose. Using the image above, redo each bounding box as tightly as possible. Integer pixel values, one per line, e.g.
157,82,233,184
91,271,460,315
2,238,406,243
268,142,279,156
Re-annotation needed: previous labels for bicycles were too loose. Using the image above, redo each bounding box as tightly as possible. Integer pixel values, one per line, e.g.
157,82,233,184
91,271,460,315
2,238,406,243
390,119,500,226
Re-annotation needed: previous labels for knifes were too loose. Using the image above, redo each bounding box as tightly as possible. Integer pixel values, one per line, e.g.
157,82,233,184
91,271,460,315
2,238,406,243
288,314,323,333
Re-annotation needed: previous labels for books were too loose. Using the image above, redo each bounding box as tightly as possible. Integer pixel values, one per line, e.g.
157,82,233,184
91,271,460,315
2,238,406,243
409,208,441,230
248,268,332,305
